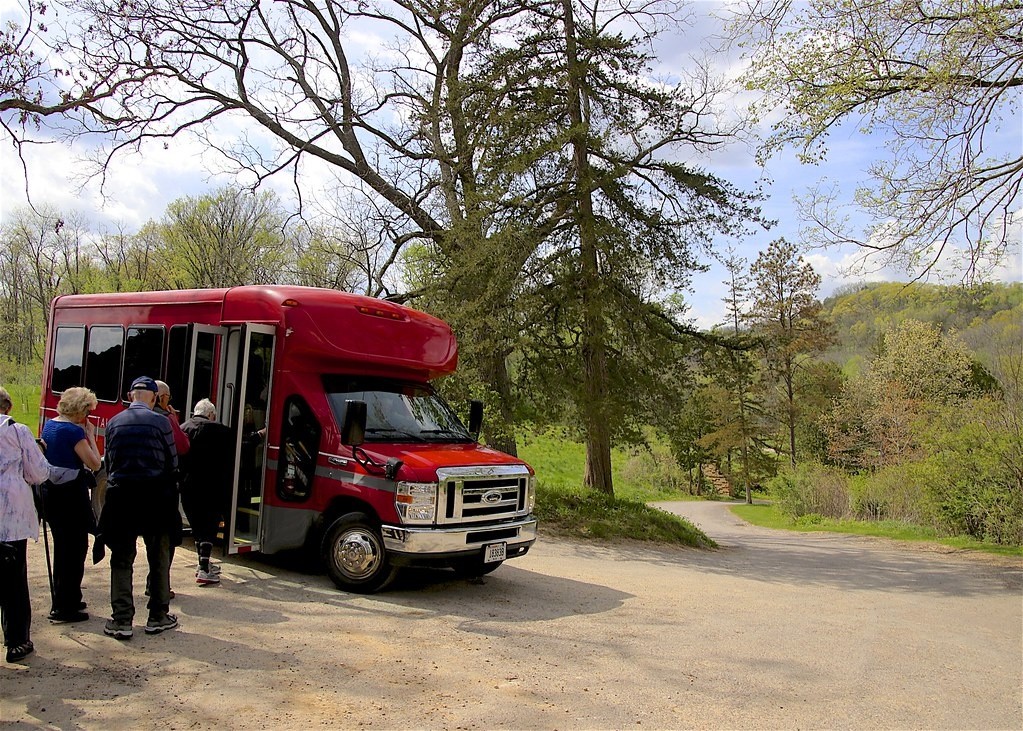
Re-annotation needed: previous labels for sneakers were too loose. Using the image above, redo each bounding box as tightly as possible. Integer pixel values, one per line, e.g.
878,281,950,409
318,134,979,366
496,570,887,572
104,620,133,640
196,570,221,583
195,562,221,577
145,614,177,636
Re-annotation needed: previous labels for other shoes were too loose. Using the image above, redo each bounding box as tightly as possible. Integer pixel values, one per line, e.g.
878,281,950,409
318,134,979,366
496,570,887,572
145,591,176,600
6,639,34,662
47,611,90,623
77,602,87,610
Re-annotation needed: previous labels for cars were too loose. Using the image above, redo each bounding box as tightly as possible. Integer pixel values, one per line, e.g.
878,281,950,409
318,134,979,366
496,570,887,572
39,280,540,591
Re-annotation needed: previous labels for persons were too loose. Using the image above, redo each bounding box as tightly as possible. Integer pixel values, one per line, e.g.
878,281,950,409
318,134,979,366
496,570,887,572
37,385,101,622
104,376,191,641
175,397,267,584
0,385,50,663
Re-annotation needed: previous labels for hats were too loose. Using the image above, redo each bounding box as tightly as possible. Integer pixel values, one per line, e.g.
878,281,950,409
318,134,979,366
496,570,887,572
130,376,159,392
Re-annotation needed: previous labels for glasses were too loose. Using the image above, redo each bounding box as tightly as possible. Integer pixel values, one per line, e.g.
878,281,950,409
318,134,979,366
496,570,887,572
160,394,172,400
86,408,91,417
